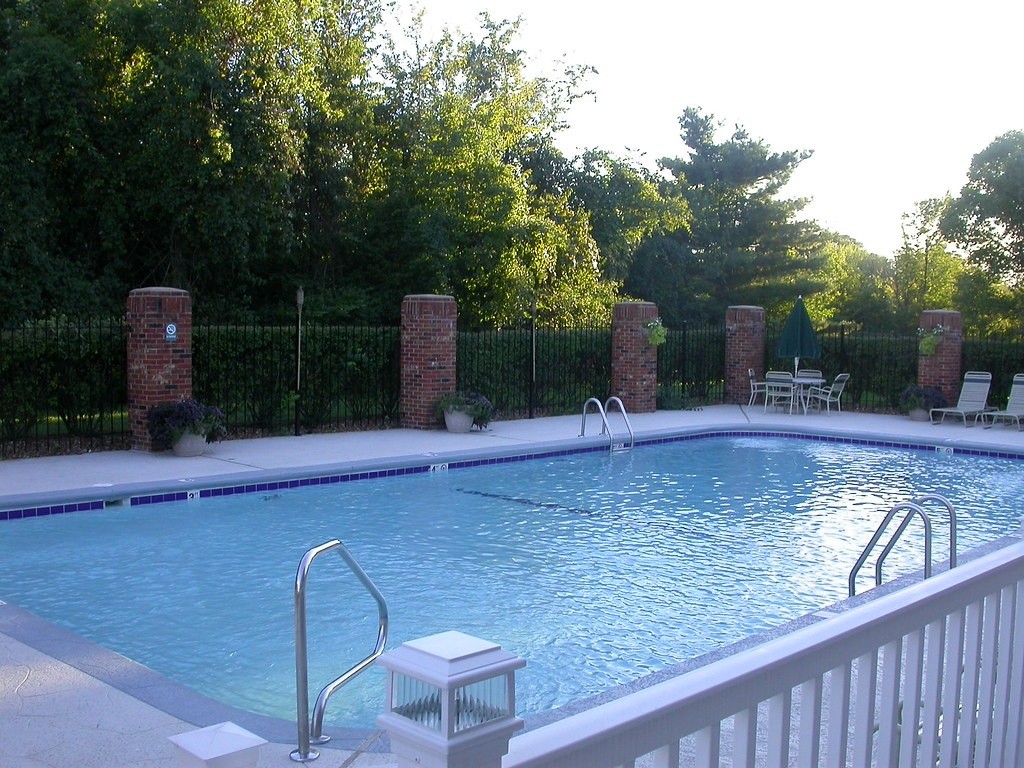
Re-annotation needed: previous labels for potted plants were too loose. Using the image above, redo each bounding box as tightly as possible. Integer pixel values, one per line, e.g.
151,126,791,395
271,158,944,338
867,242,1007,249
900,385,951,421
431,390,494,434
146,397,227,456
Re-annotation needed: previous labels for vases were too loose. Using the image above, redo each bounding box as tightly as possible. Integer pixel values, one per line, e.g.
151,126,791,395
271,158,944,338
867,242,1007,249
924,334,943,344
645,328,654,336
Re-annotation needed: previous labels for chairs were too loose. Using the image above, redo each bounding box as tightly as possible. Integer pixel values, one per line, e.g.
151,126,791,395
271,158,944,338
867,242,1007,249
795,369,823,415
981,373,1024,431
806,373,850,416
747,368,774,409
929,371,992,428
764,371,799,415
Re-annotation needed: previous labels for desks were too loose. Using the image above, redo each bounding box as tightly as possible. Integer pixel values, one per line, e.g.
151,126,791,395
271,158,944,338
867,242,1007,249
784,378,826,415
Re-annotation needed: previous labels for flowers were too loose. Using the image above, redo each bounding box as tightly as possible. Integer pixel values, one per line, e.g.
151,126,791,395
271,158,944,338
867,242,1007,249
917,323,943,334
644,317,662,327
